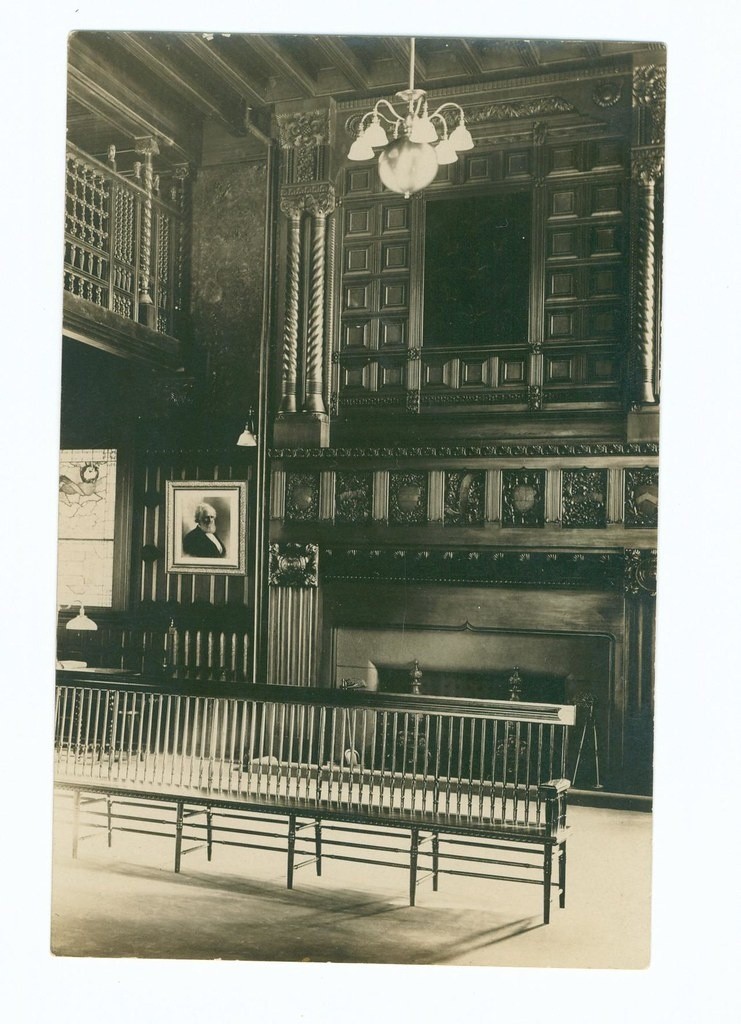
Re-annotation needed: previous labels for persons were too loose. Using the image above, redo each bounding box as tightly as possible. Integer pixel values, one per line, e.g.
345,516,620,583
180,500,227,557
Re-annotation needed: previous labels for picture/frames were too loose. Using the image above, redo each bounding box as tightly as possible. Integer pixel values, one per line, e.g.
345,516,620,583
164,480,248,577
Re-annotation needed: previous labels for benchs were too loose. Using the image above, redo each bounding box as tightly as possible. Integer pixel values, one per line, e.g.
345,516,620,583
55,667,577,925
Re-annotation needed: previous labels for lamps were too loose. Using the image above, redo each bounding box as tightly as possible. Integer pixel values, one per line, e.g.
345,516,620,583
57,598,99,631
345,35,475,201
